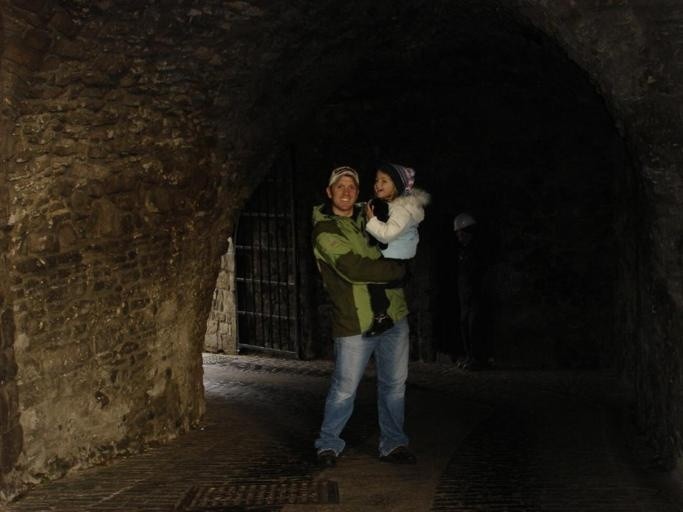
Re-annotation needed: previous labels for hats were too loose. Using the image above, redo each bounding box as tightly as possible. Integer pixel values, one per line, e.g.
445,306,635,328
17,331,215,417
328,166,359,186
380,164,414,195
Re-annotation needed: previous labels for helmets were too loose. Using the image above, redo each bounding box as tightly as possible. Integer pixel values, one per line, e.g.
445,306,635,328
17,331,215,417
454,212,476,232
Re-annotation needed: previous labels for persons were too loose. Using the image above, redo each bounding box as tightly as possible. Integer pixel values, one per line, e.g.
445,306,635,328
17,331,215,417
360,165,428,341
308,164,426,467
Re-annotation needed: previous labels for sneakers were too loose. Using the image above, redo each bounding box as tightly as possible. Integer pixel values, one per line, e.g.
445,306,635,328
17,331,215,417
317,450,337,469
378,446,416,463
366,313,395,338
